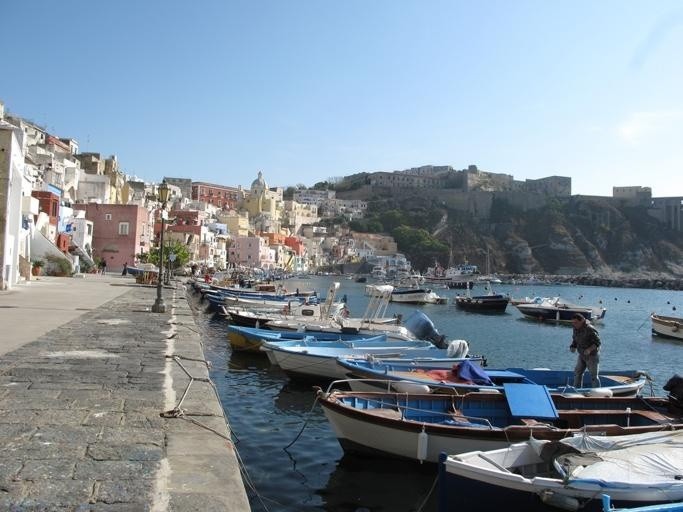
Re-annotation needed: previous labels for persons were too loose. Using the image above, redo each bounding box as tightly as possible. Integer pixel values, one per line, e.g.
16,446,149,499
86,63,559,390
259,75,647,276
121,261,127,276
100,257,106,275
568,311,602,388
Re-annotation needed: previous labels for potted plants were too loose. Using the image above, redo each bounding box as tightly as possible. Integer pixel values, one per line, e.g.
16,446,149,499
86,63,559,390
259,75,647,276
31,258,42,277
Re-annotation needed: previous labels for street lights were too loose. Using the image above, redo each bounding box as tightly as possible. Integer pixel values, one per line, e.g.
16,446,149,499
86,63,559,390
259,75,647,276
166,229,172,285
152,202,169,313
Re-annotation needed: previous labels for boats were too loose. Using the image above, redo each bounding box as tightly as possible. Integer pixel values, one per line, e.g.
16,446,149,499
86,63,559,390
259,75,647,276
127,263,165,278
